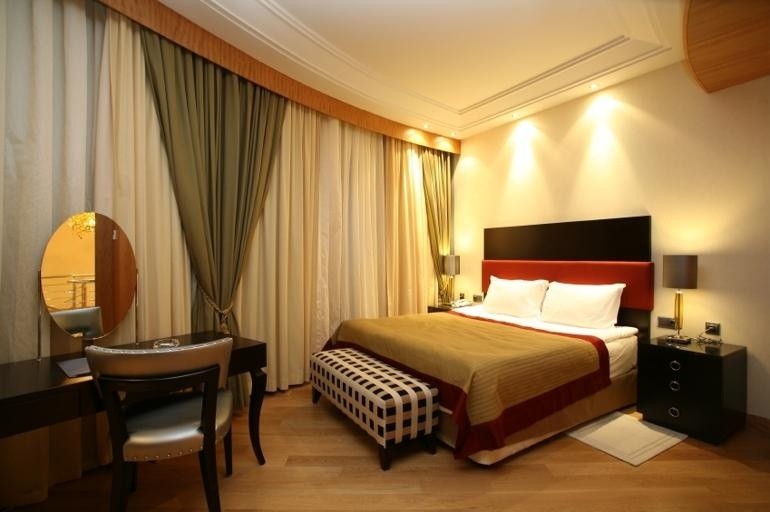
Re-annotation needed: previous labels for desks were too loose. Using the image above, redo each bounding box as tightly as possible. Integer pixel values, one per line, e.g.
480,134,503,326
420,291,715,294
0,330,268,466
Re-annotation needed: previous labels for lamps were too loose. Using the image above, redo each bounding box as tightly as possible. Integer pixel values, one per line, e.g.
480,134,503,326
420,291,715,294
69,213,95,238
663,254,698,345
440,255,460,306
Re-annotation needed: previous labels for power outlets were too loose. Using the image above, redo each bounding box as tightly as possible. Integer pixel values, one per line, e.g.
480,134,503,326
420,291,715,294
705,322,721,335
658,317,682,329
459,293,464,299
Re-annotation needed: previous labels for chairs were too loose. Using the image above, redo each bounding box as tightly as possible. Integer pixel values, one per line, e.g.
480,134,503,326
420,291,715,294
50,306,103,337
84,337,234,512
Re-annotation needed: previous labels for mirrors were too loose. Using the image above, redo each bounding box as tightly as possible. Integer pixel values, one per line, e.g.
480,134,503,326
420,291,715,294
37,212,140,363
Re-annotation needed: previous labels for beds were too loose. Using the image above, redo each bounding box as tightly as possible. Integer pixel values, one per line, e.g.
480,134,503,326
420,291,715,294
340,214,654,465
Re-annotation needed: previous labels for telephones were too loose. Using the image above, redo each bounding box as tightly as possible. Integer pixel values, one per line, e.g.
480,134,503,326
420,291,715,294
452,298,472,307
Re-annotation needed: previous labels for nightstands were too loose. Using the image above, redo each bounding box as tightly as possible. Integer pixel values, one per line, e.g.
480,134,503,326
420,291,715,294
636,336,747,446
427,304,452,312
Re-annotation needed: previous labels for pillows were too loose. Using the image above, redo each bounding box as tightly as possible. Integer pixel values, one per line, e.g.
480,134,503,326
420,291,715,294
541,281,627,329
479,273,549,321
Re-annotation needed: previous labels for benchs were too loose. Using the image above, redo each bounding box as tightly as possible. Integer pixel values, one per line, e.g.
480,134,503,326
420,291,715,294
309,348,441,471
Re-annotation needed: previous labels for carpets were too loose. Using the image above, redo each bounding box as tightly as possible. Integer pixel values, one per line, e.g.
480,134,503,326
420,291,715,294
564,412,689,466
